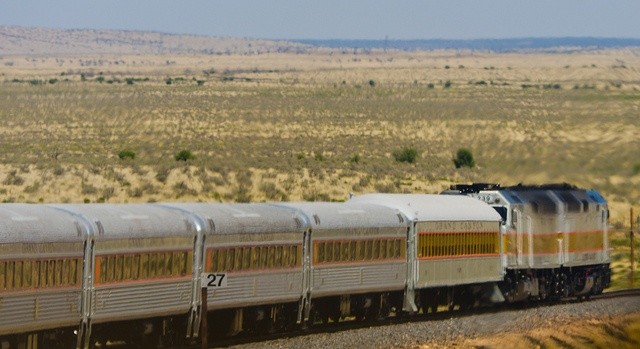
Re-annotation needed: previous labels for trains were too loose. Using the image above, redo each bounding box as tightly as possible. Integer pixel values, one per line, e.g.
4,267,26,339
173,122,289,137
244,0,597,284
0,183,612,348
